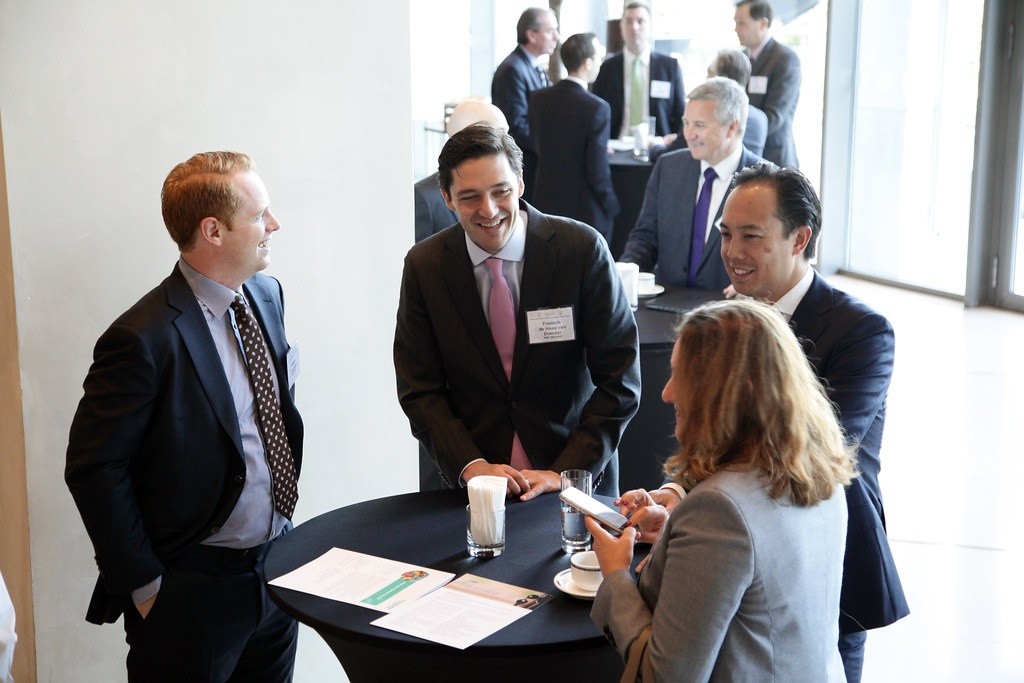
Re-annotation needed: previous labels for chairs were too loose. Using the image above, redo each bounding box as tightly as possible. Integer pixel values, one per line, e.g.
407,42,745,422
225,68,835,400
615,340,682,498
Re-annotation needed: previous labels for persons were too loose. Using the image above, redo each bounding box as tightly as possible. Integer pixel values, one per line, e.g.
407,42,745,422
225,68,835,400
64,151,303,683
414,5,617,249
583,299,857,683
618,76,780,298
393,123,641,500
648,163,910,683
598,0,800,171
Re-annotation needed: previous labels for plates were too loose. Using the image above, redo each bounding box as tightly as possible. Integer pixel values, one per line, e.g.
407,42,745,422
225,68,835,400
612,144,633,152
638,284,664,297
553,569,605,601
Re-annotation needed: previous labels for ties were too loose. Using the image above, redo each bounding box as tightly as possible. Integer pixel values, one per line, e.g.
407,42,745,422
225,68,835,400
628,57,643,137
688,168,718,284
537,63,547,87
231,292,299,521
482,258,531,472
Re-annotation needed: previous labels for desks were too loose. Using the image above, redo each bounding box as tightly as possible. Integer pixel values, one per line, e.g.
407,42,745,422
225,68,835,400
610,150,653,261
265,486,653,683
619,285,725,314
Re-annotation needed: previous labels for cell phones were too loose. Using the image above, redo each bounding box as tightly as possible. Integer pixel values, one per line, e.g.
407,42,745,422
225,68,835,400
559,486,629,533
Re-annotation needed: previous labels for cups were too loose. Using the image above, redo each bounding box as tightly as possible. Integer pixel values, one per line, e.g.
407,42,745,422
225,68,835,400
560,469,593,553
571,551,604,592
624,283,640,312
639,272,656,292
465,504,507,558
633,139,650,163
642,116,656,137
